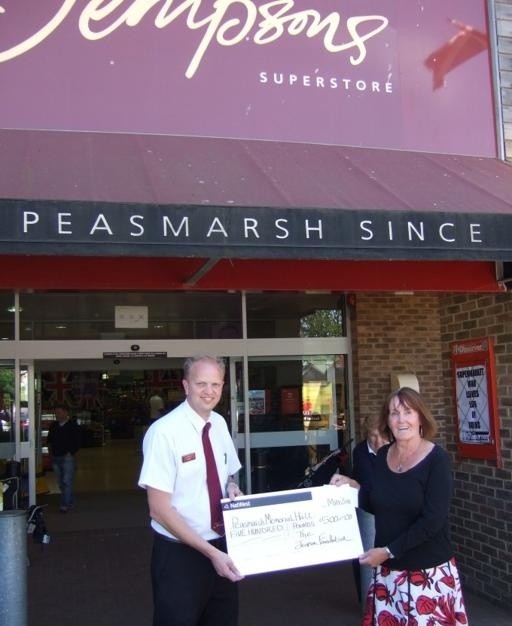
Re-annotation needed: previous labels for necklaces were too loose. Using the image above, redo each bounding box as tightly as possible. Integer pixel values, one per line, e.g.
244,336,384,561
394,438,425,474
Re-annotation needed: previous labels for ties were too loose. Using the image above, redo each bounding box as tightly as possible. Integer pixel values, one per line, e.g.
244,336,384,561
201,421,227,536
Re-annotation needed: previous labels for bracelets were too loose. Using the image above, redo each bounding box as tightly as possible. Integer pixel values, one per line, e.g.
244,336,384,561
384,546,395,559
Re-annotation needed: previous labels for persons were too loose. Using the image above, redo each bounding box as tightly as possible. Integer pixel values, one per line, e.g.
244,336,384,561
148,388,167,421
325,386,471,626
136,352,247,625
46,402,82,513
349,412,395,616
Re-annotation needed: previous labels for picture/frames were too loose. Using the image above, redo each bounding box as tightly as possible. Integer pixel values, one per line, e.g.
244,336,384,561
447,335,500,470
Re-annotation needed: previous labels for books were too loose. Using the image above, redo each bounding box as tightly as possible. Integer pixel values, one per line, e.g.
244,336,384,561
292,452,344,490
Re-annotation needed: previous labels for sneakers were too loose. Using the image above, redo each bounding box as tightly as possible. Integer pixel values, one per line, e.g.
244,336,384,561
59,501,75,511
